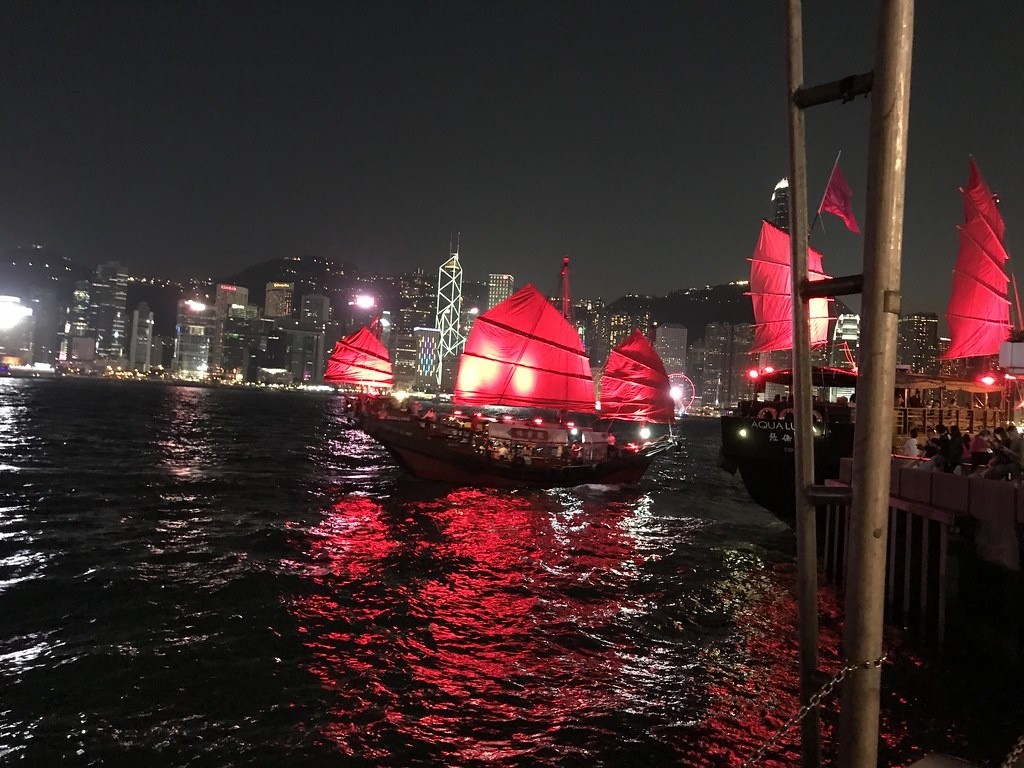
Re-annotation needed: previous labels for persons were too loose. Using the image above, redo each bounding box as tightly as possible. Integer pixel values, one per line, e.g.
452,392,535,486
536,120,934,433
423,406,436,418
486,440,582,465
770,387,1024,481
606,431,615,460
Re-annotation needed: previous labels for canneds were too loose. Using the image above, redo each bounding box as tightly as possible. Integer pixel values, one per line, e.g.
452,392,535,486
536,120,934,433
1006,472,1012,481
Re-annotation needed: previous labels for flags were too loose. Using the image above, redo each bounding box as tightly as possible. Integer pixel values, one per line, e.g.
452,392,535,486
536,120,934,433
819,164,863,235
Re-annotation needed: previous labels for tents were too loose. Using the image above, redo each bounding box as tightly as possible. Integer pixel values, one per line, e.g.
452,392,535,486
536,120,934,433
752,366,1011,408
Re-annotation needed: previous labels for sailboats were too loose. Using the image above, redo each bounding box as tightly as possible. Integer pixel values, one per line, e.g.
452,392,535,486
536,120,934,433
720,154,1024,568
323,283,680,483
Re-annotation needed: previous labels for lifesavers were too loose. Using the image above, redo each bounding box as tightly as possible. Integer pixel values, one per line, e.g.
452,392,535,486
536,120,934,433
812,410,823,423
756,407,777,420
778,408,794,420
